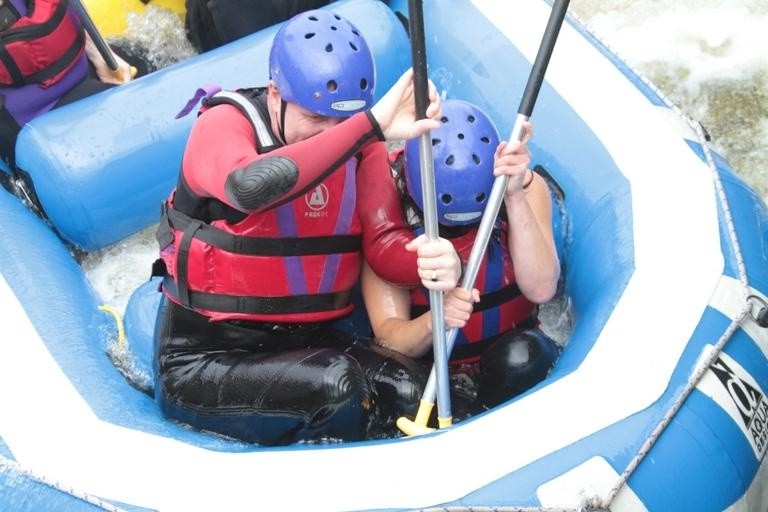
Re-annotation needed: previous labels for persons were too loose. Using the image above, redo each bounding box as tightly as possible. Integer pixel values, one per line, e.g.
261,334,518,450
0,1,153,186
356,97,563,428
148,8,464,446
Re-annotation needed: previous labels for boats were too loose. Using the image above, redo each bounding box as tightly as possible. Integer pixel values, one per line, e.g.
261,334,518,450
0,0,768,512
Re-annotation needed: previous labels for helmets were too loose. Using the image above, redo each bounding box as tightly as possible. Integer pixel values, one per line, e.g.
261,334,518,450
404,99,503,226
269,8,374,117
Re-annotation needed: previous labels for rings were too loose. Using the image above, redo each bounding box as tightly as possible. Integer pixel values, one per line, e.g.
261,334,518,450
431,269,438,282
